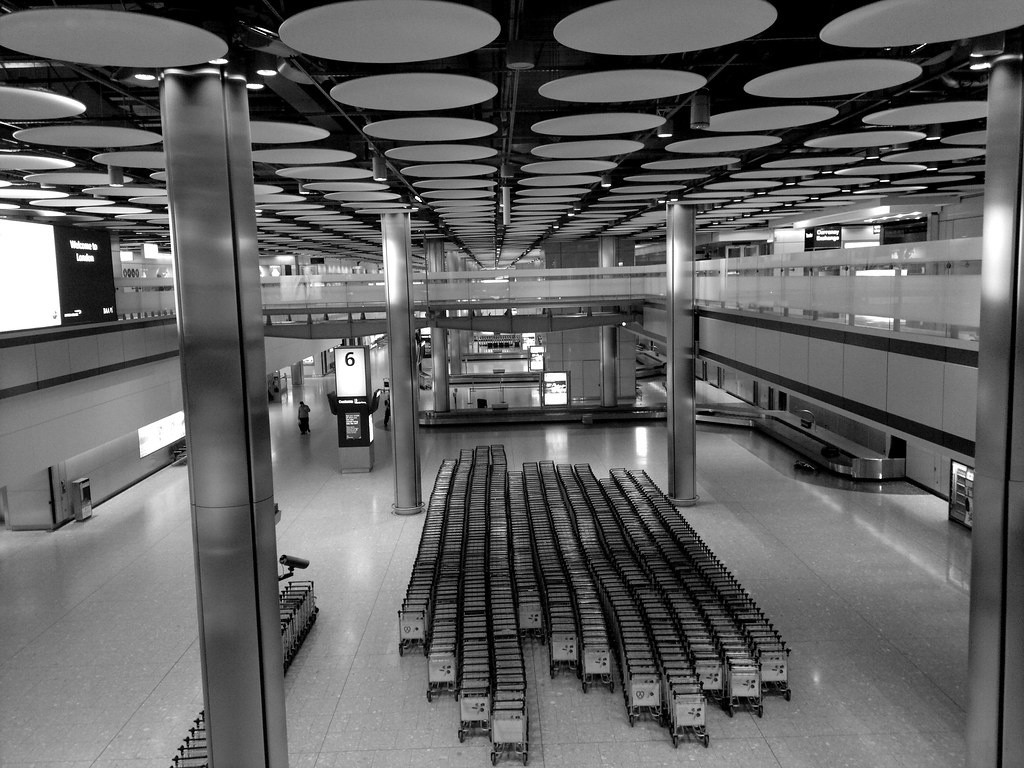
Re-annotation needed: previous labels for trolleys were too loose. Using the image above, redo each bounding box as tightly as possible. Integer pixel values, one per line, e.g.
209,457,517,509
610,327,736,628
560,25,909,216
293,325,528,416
169,582,317,768
397,443,794,766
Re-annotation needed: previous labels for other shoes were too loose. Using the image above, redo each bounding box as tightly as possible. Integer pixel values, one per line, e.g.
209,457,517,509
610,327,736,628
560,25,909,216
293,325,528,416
301,433,305,434
384,424,387,427
308,429,310,432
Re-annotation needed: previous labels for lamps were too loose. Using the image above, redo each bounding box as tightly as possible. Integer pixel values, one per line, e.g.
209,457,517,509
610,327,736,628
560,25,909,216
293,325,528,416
574,203,581,211
866,147,879,160
372,156,389,182
926,124,941,141
254,51,278,77
501,165,514,178
926,162,938,171
601,175,611,188
657,120,673,138
134,68,156,81
567,209,576,217
690,96,710,129
505,39,536,71
786,177,796,186
727,162,741,170
968,57,991,70
245,71,264,90
977,32,1005,56
298,183,310,195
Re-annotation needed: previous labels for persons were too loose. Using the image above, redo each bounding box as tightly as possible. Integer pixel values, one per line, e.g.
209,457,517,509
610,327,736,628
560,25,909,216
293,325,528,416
384,394,390,430
297,401,311,435
